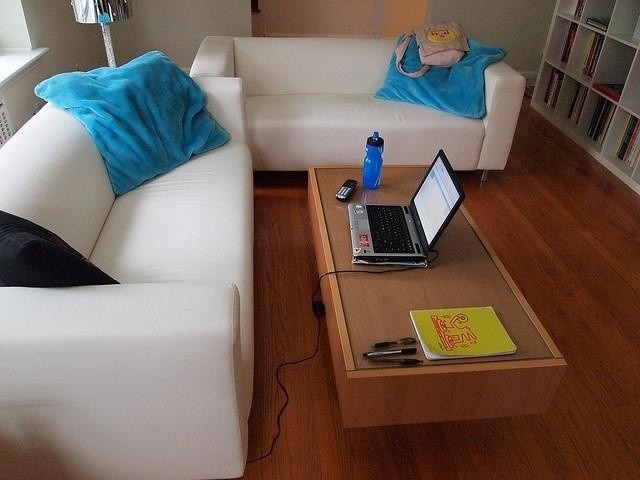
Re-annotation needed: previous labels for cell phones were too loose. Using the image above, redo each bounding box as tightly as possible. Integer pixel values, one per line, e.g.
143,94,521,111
336,178,357,200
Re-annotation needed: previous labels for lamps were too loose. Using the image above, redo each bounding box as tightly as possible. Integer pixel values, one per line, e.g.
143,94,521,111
69,0,132,68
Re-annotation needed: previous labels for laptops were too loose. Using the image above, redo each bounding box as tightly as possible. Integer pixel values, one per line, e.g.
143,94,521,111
348,148,466,263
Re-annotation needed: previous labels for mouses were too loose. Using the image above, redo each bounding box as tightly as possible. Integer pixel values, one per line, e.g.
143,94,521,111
312,300,325,317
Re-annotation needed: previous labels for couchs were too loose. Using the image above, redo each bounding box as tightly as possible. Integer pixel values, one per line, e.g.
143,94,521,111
0,73,255,478
187,37,528,184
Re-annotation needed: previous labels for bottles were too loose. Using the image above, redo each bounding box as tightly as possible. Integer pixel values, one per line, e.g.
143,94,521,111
362,131,383,188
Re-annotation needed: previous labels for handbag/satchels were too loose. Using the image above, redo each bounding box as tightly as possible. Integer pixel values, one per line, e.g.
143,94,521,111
394,21,470,79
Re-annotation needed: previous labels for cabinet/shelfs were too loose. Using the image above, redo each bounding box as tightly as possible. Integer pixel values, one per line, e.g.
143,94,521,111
530,2,640,196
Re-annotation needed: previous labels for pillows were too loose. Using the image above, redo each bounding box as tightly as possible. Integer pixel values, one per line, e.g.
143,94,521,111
376,35,508,118
34,50,230,196
0,208,121,289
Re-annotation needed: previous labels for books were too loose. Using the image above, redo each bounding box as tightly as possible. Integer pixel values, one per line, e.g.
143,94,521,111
543,0,640,168
409,307,517,361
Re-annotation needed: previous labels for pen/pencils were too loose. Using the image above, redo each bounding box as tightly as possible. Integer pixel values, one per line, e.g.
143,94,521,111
363,337,423,364
